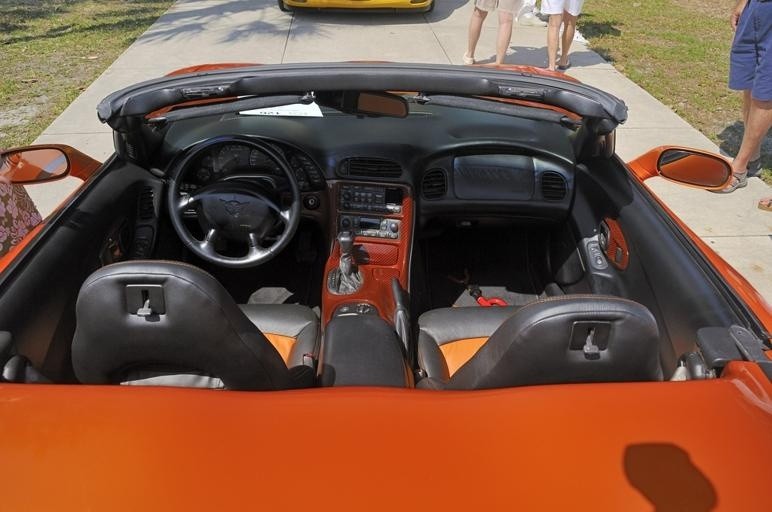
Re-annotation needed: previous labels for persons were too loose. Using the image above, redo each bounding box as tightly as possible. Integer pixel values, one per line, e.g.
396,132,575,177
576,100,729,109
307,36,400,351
718,1,772,192
540,0,583,71
463,1,523,65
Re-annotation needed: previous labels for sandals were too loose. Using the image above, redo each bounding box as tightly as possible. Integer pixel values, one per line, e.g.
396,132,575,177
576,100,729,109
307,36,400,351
758,199,772,211
705,169,748,193
746,156,763,177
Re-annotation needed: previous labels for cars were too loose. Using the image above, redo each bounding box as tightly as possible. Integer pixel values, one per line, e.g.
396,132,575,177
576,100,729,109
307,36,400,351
278,1,436,18
1,59,771,512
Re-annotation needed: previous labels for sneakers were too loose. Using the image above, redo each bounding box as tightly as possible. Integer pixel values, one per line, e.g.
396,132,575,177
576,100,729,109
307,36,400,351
559,59,570,70
462,52,475,64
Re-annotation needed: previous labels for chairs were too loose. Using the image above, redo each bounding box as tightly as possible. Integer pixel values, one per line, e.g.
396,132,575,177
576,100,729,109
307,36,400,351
417,294,664,389
70,257,320,388
